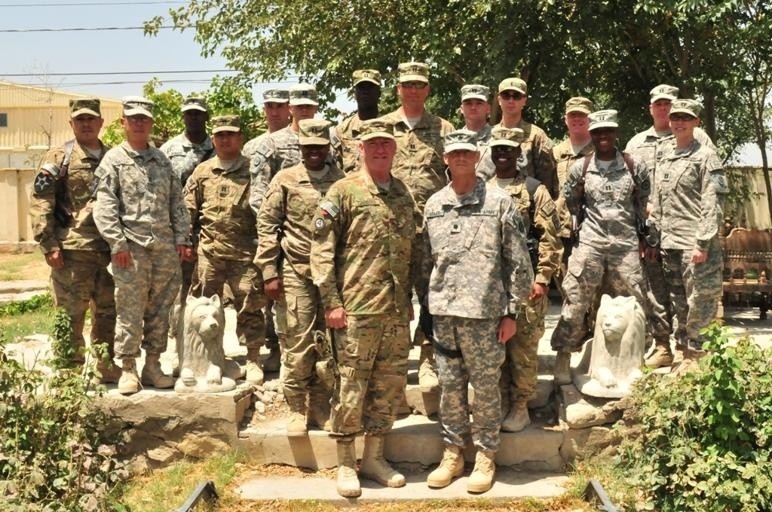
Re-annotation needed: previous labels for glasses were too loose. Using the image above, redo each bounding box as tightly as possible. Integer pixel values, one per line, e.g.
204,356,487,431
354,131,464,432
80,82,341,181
400,81,429,90
669,113,694,122
592,128,615,135
72,116,98,122
500,92,526,101
294,104,314,109
493,146,515,153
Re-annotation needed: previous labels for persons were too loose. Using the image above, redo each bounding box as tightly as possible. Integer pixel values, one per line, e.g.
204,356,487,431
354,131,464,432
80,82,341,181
28,96,123,385
157,96,218,374
180,115,266,385
554,96,594,181
649,96,730,375
551,109,653,384
484,127,564,432
456,84,529,179
419,128,535,493
87,96,188,396
250,82,321,217
242,87,291,159
334,68,386,174
357,62,457,393
625,84,717,367
253,118,334,437
311,119,423,497
488,76,561,203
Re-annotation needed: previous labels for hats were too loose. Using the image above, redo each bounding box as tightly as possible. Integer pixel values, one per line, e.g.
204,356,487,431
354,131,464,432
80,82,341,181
668,99,702,119
122,95,154,119
297,118,332,146
262,88,290,104
444,128,480,154
397,62,429,84
649,84,680,104
564,96,592,115
586,110,619,132
69,98,101,119
212,114,241,134
359,120,395,141
289,84,320,106
497,77,528,95
352,69,381,87
460,84,489,102
181,97,207,112
488,127,525,148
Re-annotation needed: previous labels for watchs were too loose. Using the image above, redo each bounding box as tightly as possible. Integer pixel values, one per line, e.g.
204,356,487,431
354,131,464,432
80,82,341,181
506,313,520,319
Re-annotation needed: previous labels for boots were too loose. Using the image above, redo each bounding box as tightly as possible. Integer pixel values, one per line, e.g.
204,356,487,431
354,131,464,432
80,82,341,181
500,394,511,419
645,338,674,369
117,357,144,395
671,346,687,365
140,353,175,389
417,345,440,393
285,393,308,437
263,343,281,371
467,445,496,494
336,440,362,498
553,349,573,385
501,400,531,433
673,346,705,375
306,391,333,431
358,434,406,488
426,443,465,488
94,358,123,385
245,346,265,384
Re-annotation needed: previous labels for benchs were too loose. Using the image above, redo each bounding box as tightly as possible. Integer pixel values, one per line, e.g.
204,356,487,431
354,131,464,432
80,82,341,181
723,227,772,320
